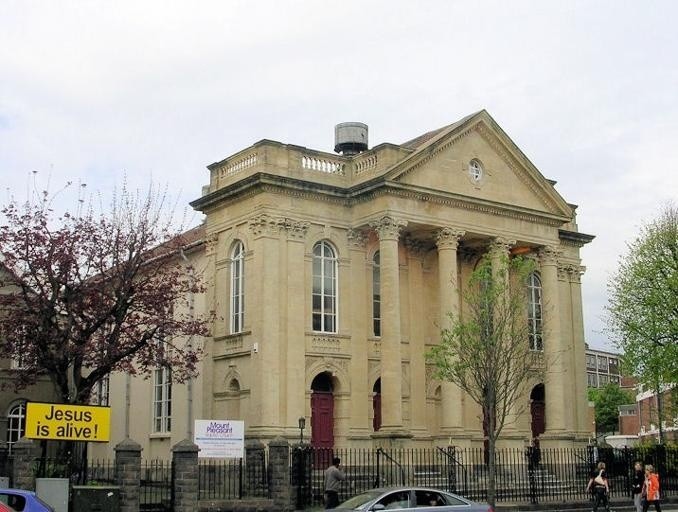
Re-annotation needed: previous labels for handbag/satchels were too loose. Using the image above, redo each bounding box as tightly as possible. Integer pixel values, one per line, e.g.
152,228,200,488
594,470,604,486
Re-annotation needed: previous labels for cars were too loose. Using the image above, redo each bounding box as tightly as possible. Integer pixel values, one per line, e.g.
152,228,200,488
321,485,495,512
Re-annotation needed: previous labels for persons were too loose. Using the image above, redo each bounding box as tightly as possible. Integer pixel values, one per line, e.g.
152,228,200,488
584,461,611,511
630,460,648,511
639,464,662,511
428,494,439,506
523,439,543,472
322,457,347,509
